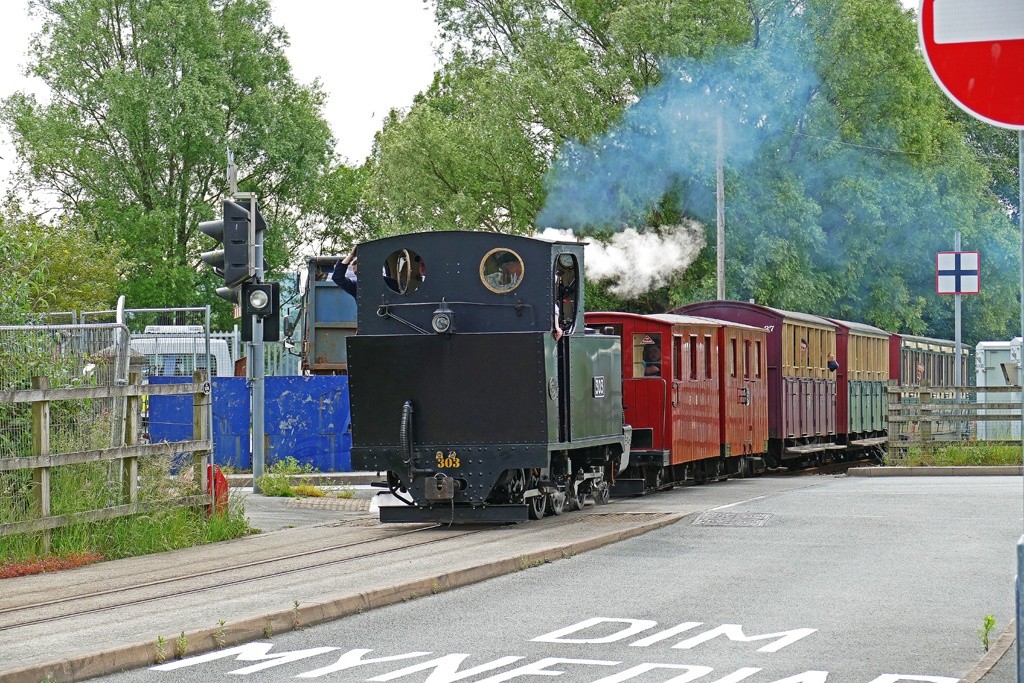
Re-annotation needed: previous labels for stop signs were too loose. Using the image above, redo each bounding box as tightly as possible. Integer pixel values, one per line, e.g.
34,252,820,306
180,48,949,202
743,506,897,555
918,0,1024,131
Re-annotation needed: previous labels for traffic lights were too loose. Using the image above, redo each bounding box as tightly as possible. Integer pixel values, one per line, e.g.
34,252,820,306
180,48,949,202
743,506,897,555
209,264,243,319
198,199,255,287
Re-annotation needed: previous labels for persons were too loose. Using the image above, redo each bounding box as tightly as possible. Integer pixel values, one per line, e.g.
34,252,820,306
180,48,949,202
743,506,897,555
414,255,426,282
332,246,406,305
916,363,924,379
486,252,521,289
644,339,661,376
827,353,839,372
801,338,808,354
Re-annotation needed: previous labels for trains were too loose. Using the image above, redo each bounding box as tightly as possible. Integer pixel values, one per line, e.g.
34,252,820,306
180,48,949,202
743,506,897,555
344,230,971,530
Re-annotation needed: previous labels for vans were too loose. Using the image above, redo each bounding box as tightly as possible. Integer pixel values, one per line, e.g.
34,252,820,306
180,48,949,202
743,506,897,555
72,333,234,392
